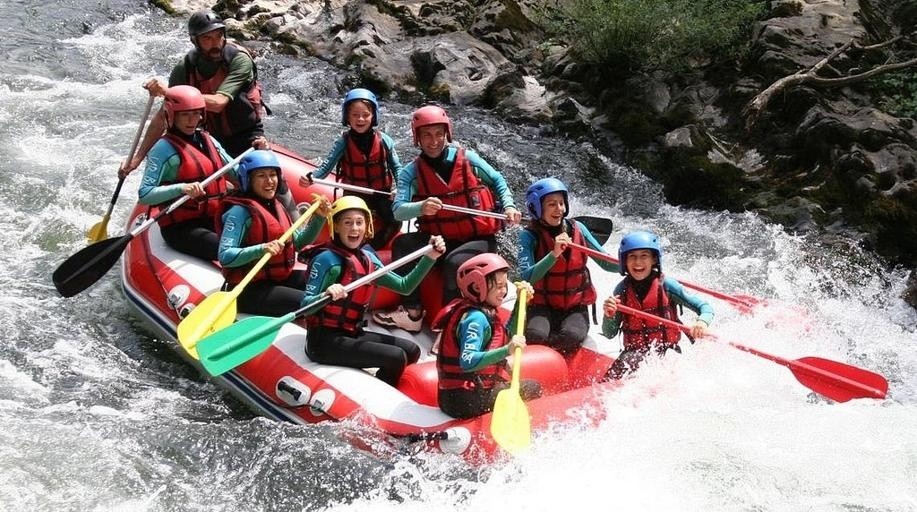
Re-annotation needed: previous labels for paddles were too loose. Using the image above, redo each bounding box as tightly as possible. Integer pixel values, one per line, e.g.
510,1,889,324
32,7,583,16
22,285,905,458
607,299,888,403
195,237,443,378
560,243,768,315
177,192,321,361
489,281,535,455
53,83,162,243
52,138,259,298
442,203,612,247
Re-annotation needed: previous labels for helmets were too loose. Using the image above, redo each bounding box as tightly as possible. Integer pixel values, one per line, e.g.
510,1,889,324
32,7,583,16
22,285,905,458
411,105,453,147
162,85,208,131
188,10,227,48
456,252,511,304
327,196,374,240
618,232,664,277
238,150,283,194
340,88,381,127
526,178,570,220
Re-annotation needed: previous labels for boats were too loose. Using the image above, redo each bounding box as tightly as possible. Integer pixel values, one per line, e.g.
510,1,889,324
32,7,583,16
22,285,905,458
114,138,660,467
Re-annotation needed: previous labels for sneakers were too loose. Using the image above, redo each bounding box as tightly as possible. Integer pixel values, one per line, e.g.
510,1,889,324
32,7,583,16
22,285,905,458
372,305,426,335
431,329,443,354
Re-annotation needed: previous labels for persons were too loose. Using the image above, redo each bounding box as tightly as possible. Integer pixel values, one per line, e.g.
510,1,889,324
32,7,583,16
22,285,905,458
116,11,262,179
298,89,403,252
138,85,267,260
389,106,522,307
601,232,715,384
518,178,622,357
303,195,446,388
436,253,542,419
217,150,330,320
